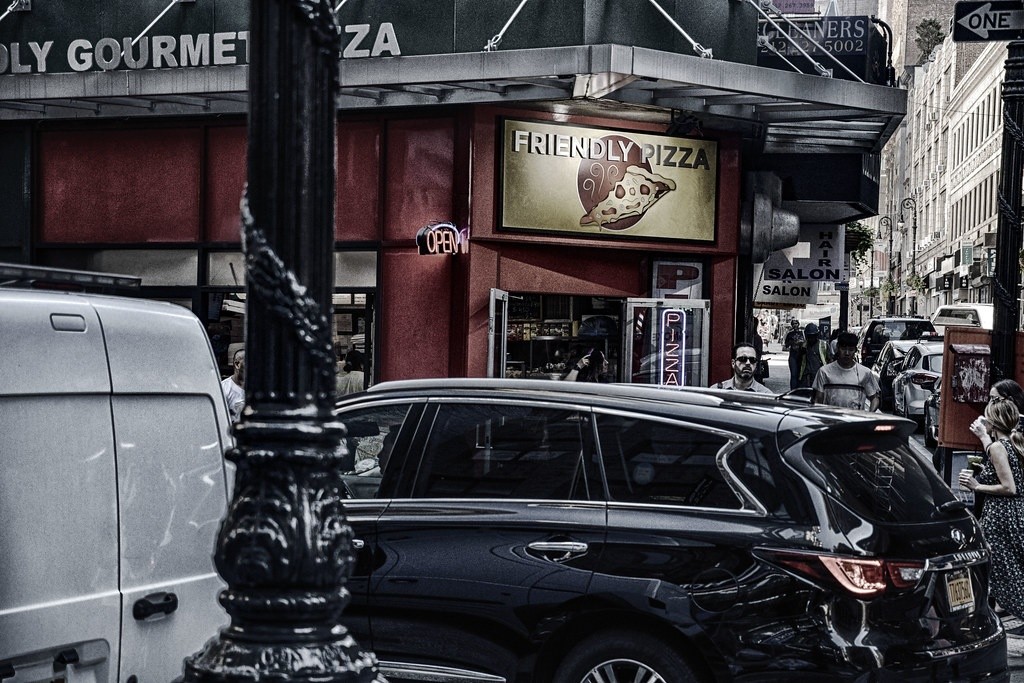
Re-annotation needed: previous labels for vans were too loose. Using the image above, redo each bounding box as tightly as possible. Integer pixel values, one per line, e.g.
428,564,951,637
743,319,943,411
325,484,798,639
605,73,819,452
922,300,997,339
0,261,238,683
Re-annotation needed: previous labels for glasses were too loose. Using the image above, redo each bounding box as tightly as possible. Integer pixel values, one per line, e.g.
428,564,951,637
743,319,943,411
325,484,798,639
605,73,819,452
735,356,758,364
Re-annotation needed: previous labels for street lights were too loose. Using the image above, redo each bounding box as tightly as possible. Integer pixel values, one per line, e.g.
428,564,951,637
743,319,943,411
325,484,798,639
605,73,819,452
897,198,918,319
876,215,894,318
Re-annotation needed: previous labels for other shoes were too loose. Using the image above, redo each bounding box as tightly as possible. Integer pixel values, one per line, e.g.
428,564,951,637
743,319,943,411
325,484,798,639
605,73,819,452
992,609,1010,618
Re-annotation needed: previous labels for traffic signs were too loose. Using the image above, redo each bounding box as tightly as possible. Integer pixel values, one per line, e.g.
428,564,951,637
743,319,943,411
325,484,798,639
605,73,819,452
951,1,1023,45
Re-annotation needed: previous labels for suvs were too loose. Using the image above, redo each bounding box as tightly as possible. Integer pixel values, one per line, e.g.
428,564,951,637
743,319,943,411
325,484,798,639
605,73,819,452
329,377,1012,683
854,316,939,369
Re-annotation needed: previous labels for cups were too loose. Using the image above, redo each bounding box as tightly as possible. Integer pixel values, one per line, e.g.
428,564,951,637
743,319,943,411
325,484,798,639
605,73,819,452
967,455,983,475
978,415,994,433
959,469,974,491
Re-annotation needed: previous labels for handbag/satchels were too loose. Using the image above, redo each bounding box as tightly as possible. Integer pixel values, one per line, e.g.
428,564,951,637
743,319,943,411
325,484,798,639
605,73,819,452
754,360,769,378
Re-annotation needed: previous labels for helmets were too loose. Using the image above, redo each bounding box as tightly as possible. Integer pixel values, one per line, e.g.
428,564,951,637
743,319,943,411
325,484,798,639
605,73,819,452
804,323,818,334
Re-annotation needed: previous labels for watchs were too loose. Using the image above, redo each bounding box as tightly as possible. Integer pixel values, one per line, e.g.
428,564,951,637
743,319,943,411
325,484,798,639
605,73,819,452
574,364,581,371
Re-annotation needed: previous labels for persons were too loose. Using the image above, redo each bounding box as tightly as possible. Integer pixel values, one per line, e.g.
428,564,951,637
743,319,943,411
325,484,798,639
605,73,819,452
813,334,881,412
785,320,805,390
959,380,1024,623
763,310,774,343
800,323,841,388
710,343,774,394
337,350,371,396
562,348,609,383
221,349,246,423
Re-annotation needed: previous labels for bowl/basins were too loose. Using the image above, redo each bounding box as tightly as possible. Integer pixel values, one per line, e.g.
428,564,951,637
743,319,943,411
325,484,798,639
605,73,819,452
547,373,562,379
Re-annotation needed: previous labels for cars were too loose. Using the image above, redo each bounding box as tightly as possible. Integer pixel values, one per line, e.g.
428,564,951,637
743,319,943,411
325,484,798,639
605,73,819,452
890,342,946,421
921,379,942,450
865,340,929,415
